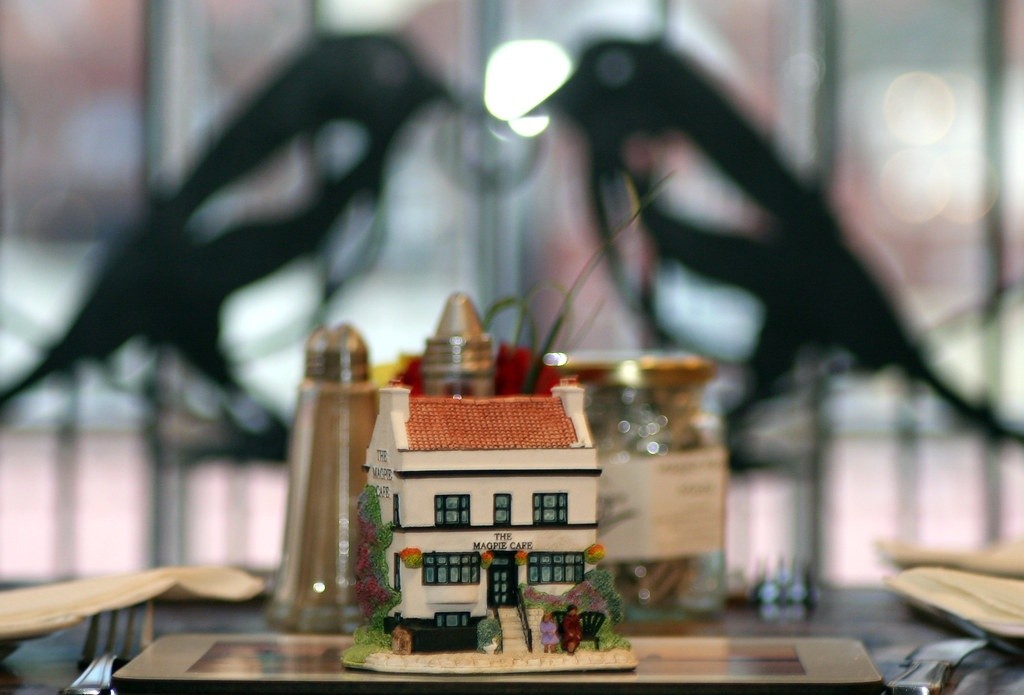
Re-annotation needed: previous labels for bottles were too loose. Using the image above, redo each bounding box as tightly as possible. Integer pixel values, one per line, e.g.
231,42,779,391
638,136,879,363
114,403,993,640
423,296,495,402
556,355,730,625
269,324,379,636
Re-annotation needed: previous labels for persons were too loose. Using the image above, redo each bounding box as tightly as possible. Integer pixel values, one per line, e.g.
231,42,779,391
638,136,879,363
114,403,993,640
561,604,582,655
539,613,559,654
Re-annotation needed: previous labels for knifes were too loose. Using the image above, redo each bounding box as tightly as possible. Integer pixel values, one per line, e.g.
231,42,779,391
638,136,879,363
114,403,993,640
884,637,988,695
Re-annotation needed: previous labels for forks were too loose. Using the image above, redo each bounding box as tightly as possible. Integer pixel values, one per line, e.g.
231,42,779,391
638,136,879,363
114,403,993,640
60,598,155,695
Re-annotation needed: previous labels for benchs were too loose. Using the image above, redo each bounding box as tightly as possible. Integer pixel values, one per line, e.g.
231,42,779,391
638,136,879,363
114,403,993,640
883,564,1024,694
0,556,264,695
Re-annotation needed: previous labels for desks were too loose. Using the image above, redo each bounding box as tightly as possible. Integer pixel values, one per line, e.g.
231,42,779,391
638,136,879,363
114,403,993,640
110,635,885,695
